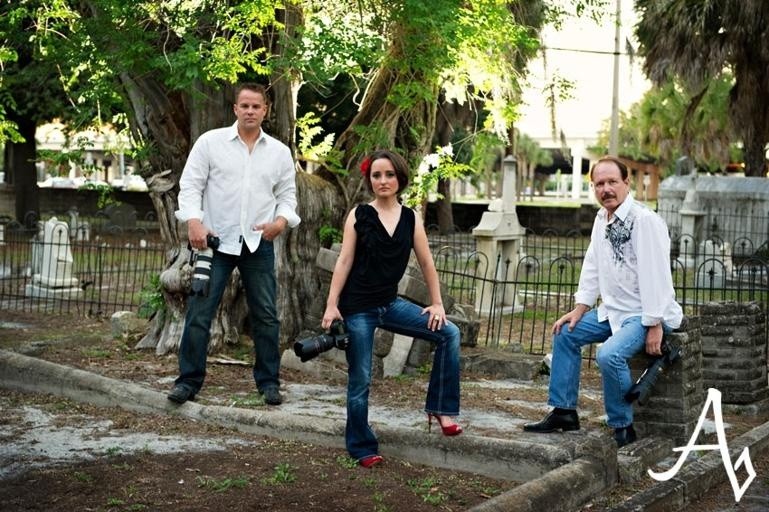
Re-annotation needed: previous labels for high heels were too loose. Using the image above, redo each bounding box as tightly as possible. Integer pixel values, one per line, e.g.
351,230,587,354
360,455,383,467
428,413,462,435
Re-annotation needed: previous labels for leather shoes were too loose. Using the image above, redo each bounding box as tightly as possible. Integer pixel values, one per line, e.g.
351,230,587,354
614,425,635,447
524,408,579,433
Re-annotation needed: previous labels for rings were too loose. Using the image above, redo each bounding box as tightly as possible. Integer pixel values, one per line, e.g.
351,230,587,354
435,313,440,321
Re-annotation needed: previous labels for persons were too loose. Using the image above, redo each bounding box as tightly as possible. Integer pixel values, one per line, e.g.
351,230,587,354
320,149,465,465
522,156,683,447
166,80,302,405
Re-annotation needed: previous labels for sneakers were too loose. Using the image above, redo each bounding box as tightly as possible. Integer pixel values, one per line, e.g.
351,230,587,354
168,385,195,402
263,387,281,404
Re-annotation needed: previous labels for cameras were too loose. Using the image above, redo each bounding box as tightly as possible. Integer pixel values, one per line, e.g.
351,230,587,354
188,235,220,298
626,339,682,406
294,320,351,362
